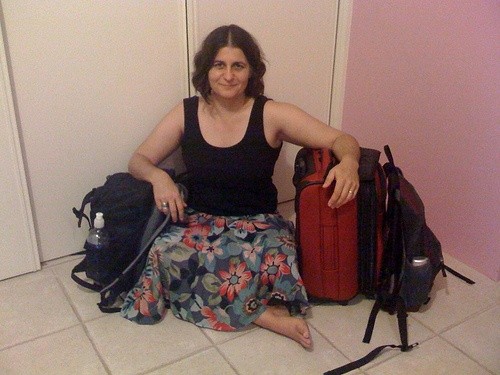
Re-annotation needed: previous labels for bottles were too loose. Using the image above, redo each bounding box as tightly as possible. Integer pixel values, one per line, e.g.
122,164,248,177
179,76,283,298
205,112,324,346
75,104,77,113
411,257,429,267
87,212,113,249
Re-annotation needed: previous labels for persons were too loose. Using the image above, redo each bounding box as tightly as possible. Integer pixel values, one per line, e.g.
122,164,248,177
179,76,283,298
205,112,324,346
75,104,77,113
128,24,361,349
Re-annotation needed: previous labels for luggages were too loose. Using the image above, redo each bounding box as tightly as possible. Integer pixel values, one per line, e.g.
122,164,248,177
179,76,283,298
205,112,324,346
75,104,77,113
295,148,387,305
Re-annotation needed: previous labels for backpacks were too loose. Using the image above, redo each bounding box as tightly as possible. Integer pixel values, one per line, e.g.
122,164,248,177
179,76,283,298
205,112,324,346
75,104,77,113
78,171,155,286
378,145,443,315
98,183,189,313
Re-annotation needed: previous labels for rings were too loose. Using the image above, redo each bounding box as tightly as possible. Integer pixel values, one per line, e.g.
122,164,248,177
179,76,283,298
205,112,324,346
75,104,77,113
349,191,353,194
161,202,168,210
